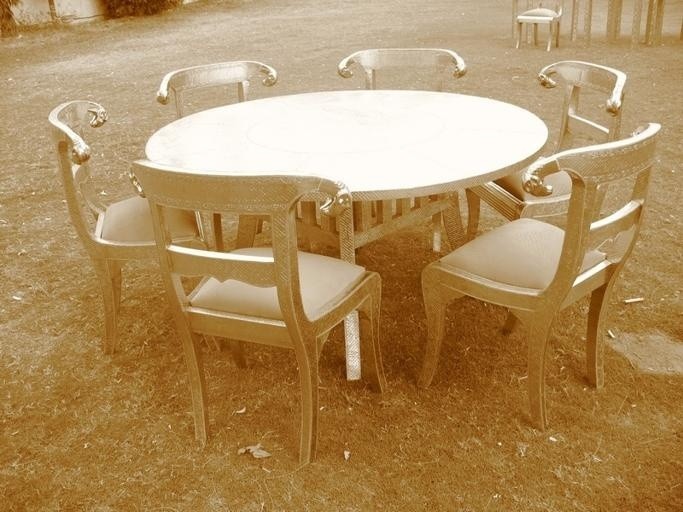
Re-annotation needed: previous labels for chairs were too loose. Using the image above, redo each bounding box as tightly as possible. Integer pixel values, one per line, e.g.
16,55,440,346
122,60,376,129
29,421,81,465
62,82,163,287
412,119,662,431
47,100,223,354
465,57,628,334
515,0,563,52
156,61,278,251
337,47,467,253
125,160,388,471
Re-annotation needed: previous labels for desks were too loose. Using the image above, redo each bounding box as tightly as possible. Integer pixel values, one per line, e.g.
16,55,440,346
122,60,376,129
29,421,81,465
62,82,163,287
143,85,549,380
